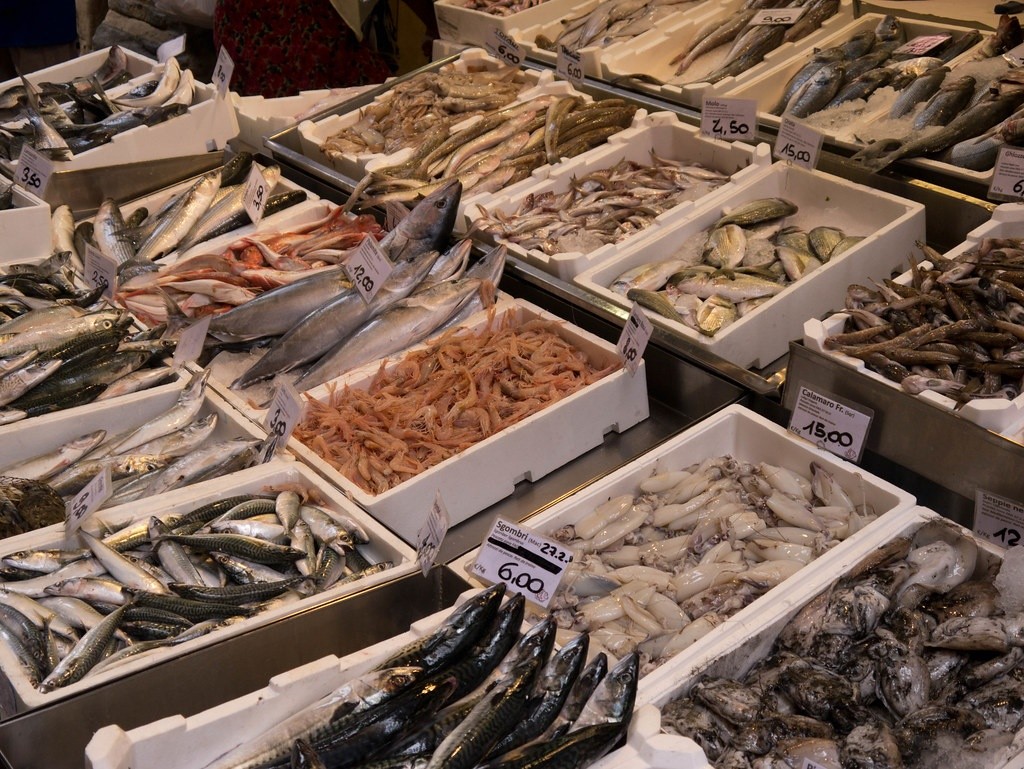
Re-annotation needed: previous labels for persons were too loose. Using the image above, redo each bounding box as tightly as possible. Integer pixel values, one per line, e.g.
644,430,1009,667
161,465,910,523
211,0,397,97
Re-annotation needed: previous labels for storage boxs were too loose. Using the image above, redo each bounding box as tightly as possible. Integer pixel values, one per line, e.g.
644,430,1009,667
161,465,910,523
0,44,240,173
102,198,389,333
298,47,554,183
0,460,427,710
0,255,193,430
433,0,1024,186
444,404,918,699
464,112,772,283
633,505,1024,769
364,78,648,232
803,203,1024,434
572,159,926,370
0,378,295,545
83,586,661,769
227,77,399,160
256,297,650,549
171,269,515,420
50,161,319,292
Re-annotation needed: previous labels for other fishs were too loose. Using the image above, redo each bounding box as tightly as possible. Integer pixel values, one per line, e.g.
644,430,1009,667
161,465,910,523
0,50,508,409
316,0,1024,410
0,369,640,769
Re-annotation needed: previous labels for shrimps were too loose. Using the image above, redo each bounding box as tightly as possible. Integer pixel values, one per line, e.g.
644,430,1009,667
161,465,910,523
290,303,625,496
